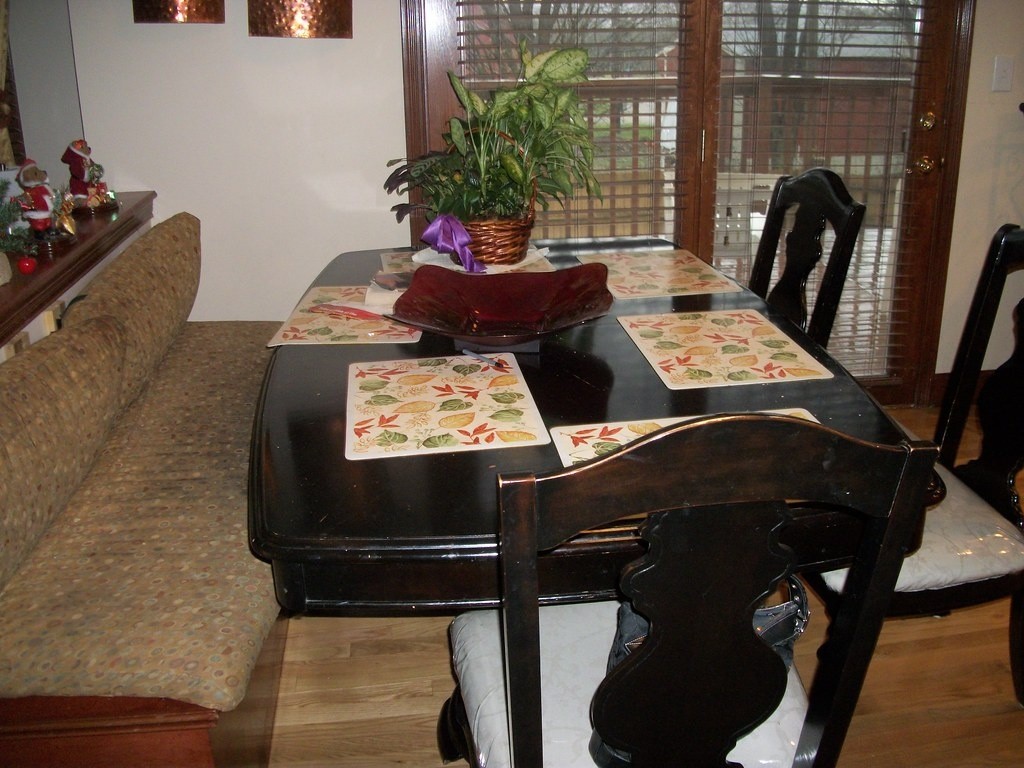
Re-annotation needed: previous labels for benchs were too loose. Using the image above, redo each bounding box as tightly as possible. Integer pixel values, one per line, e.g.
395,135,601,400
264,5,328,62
0,212,281,768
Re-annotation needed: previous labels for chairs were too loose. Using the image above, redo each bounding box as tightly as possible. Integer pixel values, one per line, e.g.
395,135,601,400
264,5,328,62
802,224,1024,710
437,413,941,768
712,175,791,293
748,167,866,350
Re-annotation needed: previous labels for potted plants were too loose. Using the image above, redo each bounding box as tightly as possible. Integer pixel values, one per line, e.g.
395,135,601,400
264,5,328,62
384,38,602,266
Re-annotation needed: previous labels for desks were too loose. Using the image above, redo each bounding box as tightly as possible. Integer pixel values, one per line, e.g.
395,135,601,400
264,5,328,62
248,234,947,760
1,190,159,367
